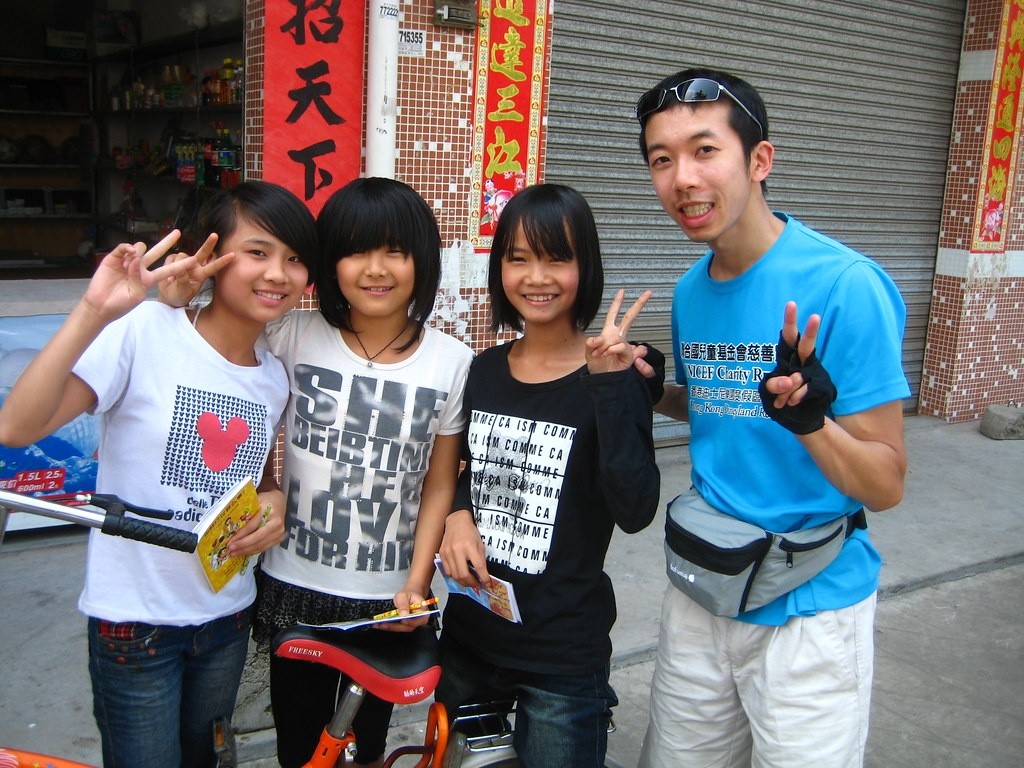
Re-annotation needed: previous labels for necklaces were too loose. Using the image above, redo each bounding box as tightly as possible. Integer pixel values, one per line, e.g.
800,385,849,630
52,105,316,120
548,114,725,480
347,318,410,367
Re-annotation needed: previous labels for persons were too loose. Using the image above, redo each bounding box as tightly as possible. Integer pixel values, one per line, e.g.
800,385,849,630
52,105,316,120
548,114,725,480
627,68,911,768
439,184,661,768
158,178,476,768
0,180,318,768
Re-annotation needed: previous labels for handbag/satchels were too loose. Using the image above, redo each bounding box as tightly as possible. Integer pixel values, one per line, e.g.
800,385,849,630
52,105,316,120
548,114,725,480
664,481,868,619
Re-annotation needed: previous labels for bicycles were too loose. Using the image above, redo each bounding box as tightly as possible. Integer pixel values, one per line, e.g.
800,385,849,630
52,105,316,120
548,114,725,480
0,485,628,768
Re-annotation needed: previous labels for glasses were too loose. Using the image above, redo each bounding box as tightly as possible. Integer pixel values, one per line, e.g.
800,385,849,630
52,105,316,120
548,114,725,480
634,78,763,141
